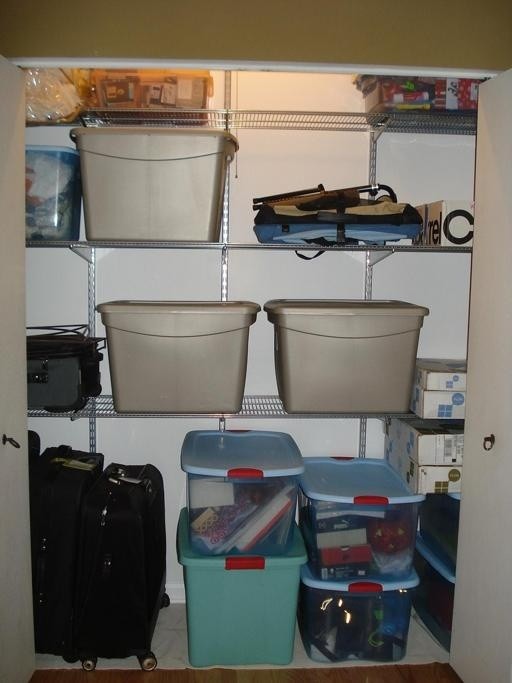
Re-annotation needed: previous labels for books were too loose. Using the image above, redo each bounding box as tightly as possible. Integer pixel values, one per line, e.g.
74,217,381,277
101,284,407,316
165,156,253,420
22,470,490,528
196,478,299,555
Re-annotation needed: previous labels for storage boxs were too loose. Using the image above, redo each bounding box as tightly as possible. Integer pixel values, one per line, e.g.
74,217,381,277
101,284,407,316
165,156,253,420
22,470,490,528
96,298,262,418
174,420,464,667
24,123,239,243
262,298,428,417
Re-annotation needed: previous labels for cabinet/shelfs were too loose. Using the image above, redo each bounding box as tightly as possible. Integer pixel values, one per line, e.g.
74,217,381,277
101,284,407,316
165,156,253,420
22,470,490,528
21,104,478,421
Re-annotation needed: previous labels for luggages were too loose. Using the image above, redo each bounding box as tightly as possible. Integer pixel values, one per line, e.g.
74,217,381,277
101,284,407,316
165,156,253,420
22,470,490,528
28,427,172,674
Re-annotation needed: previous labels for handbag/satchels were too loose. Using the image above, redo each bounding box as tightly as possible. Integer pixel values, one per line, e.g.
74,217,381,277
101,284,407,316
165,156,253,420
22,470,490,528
250,198,428,247
